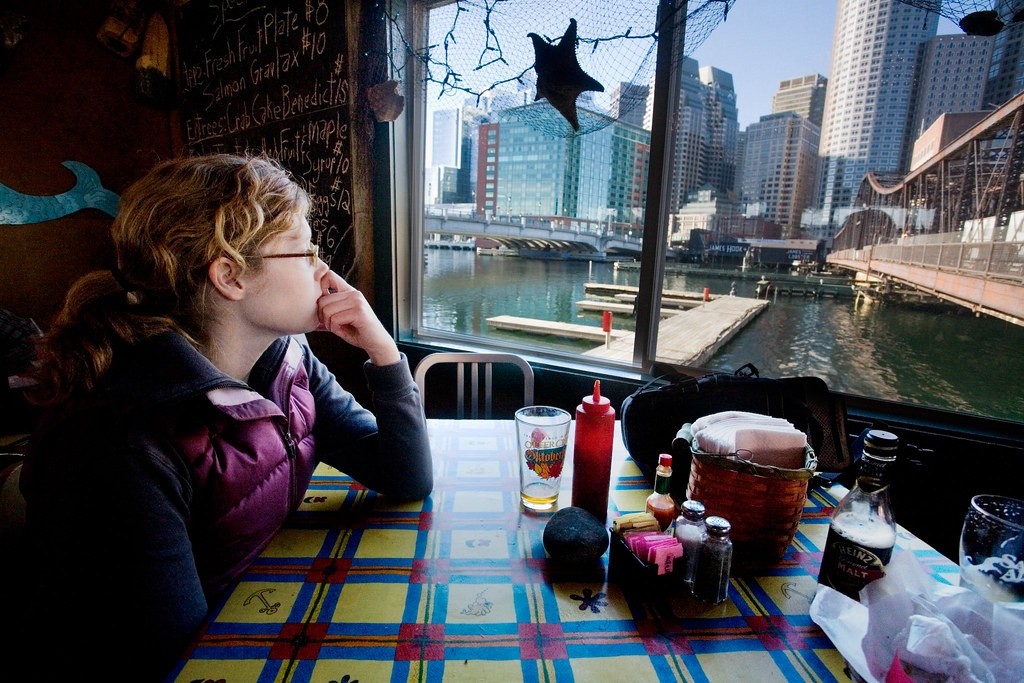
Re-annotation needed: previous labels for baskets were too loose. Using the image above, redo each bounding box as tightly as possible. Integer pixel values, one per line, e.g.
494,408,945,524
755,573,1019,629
671,410,818,567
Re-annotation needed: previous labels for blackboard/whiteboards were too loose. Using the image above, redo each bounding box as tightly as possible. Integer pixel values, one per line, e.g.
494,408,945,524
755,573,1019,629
165,0,374,306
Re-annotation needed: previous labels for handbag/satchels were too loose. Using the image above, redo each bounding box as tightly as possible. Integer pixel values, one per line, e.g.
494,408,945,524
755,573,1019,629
621,362,851,473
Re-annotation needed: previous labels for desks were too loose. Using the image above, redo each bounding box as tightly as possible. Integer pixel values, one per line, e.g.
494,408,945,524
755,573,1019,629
174,420,962,683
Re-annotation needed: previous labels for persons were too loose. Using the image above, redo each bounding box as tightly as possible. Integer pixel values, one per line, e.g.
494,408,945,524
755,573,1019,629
0,153,433,683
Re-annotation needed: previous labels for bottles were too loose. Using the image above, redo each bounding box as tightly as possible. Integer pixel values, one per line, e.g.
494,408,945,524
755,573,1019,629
817,430,899,603
572,380,615,526
646,454,675,532
693,515,734,605
674,501,706,578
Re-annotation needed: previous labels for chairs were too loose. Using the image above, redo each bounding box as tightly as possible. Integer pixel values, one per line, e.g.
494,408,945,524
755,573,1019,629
414,352,534,419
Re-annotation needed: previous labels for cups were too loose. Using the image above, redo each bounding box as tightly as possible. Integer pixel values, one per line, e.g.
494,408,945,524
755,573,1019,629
959,494,1024,604
515,406,572,509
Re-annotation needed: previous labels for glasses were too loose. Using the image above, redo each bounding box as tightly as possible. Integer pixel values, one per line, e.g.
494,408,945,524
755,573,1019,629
246,242,320,270
